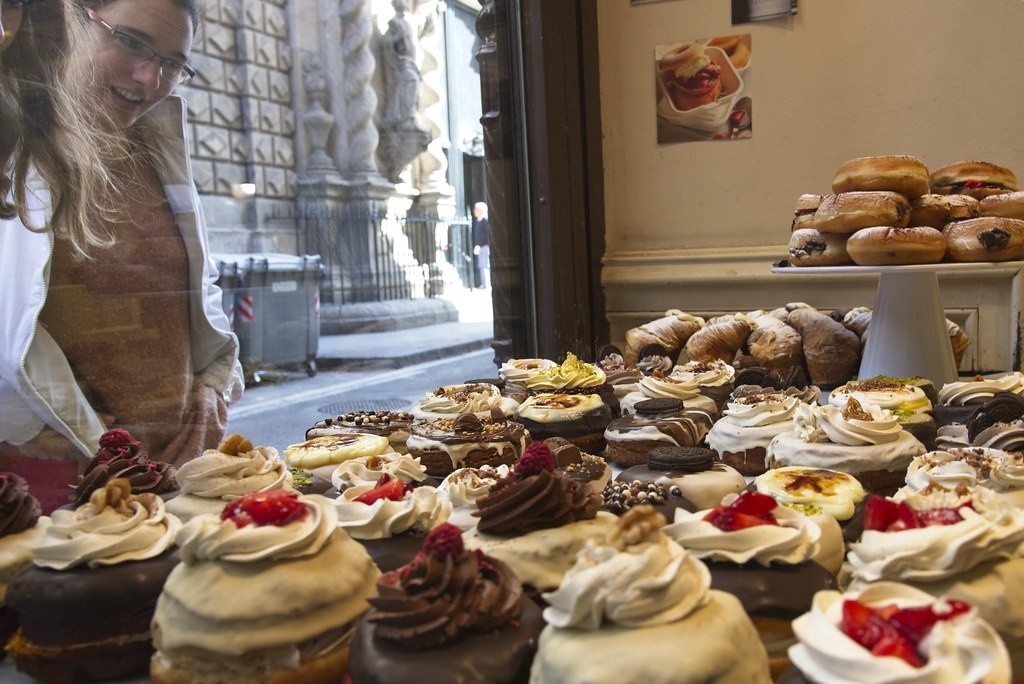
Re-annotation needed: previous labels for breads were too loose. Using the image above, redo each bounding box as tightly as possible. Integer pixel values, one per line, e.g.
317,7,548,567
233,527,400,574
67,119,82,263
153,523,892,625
624,305,970,390
785,153,1023,265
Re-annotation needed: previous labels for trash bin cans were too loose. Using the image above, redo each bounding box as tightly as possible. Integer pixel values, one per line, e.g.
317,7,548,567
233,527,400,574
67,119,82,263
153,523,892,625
213,254,262,384
236,252,322,384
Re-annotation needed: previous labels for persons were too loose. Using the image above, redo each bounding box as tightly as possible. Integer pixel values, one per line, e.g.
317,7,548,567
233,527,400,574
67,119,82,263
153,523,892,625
0,1,246,470
473,201,490,291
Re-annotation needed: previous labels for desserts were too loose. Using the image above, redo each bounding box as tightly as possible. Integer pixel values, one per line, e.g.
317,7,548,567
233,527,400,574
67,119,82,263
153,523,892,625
658,35,751,133
0,351,1024,684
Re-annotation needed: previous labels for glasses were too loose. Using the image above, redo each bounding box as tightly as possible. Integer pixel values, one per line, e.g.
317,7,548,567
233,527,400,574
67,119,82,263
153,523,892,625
78,4,197,88
